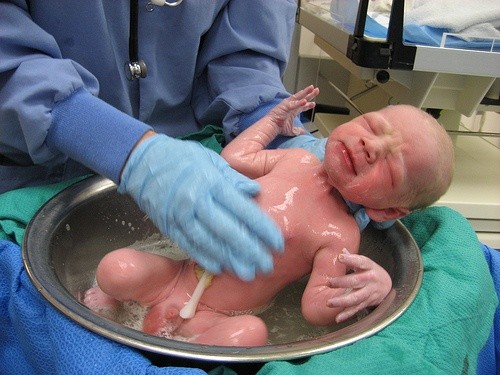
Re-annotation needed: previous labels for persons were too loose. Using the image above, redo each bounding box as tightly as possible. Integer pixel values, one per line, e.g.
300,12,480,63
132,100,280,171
0,0,400,282
83,83,456,346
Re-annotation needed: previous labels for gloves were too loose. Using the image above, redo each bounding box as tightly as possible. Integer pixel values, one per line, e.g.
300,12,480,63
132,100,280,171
278,134,397,234
117,133,280,282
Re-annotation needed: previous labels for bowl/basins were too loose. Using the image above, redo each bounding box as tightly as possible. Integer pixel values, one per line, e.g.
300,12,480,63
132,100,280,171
20,173,424,375
